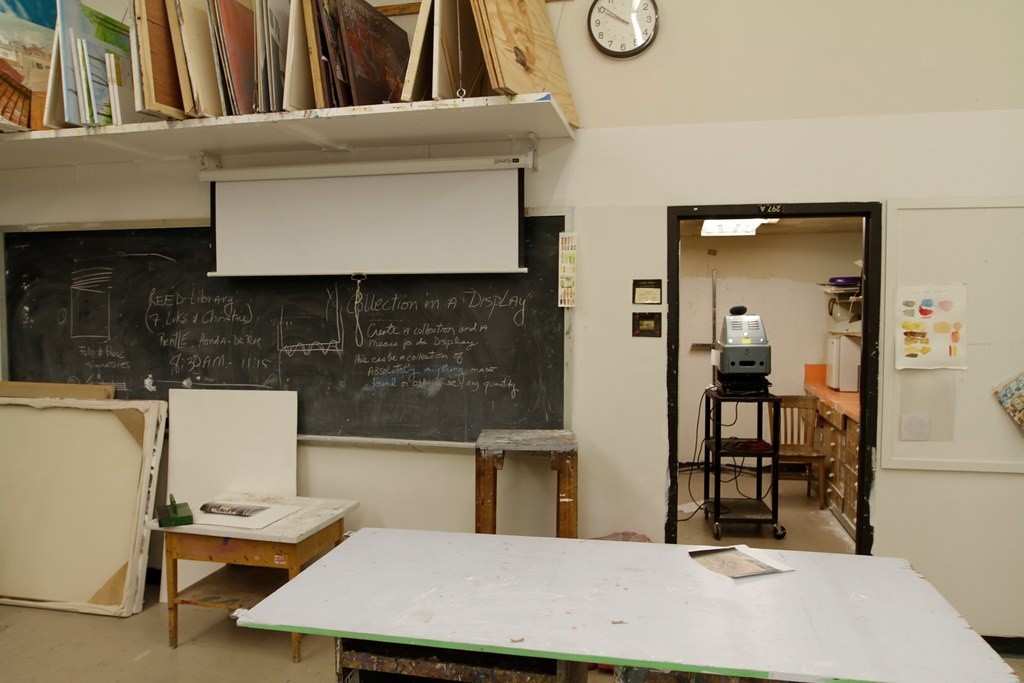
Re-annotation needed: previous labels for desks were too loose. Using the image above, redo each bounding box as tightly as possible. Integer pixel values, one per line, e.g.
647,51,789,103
146,489,360,662
231,528,1023,682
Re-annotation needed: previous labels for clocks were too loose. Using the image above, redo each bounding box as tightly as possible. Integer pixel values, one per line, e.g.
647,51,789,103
586,0,659,59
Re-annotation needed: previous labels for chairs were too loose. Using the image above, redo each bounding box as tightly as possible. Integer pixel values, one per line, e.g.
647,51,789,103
768,395,828,510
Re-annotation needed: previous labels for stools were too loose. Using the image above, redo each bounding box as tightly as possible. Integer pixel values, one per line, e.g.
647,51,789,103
475,428,579,538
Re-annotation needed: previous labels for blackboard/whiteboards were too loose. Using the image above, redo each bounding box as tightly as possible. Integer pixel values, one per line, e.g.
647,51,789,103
0,204,576,452
879,197,1024,474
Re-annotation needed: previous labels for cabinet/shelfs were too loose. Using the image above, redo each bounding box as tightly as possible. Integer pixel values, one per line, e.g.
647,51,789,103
1,91,574,173
805,384,861,541
704,387,787,541
